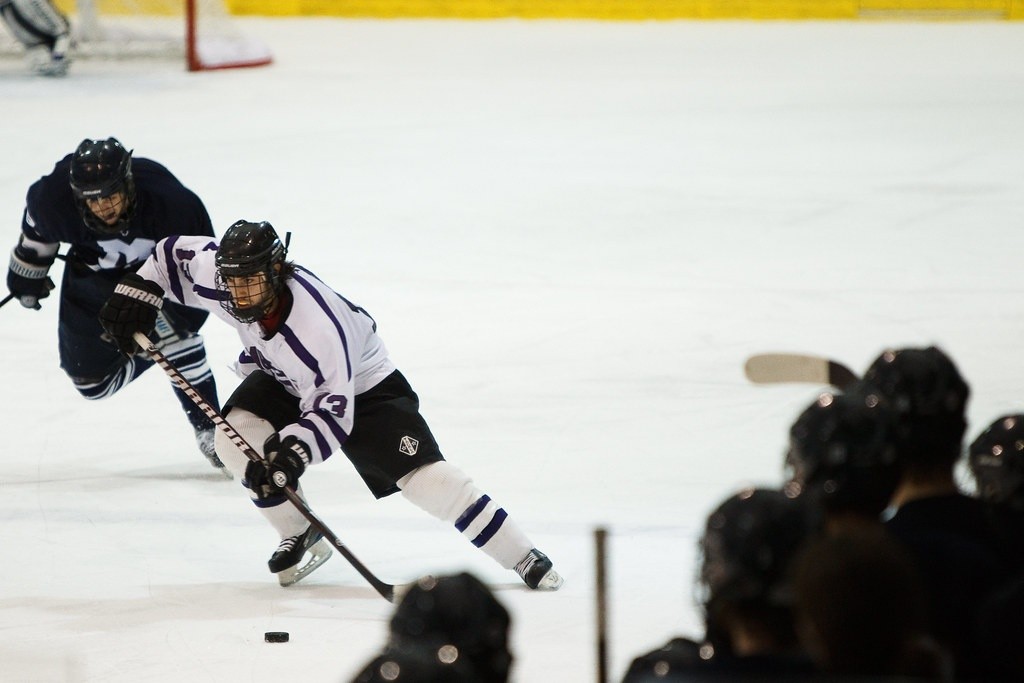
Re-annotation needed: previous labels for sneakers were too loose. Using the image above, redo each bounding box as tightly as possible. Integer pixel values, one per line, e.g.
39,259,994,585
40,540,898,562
513,548,565,590
268,524,333,584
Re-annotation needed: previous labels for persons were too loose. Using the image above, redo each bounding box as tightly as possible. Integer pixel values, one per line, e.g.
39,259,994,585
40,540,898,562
99,219,563,591
346,346,1024,683
7,137,236,480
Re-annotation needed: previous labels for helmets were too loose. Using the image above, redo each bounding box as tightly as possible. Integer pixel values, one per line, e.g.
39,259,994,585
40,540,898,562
622,346,1024,683
348,572,515,683
69,136,132,195
215,220,284,272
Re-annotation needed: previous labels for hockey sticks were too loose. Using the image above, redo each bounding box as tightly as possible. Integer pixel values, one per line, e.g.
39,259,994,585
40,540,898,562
132,331,434,603
594,527,610,683
745,351,862,390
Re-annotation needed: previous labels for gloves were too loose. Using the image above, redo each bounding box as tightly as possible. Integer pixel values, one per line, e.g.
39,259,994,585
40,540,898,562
100,273,165,354
6,246,55,311
240,434,313,508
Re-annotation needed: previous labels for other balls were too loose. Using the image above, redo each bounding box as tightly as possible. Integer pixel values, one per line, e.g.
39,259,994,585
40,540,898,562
264,631,290,643
